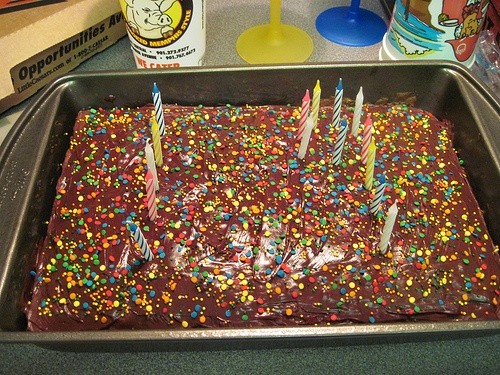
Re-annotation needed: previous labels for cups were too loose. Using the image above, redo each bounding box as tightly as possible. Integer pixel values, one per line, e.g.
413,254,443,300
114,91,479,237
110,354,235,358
378,0,490,70
118,1,206,68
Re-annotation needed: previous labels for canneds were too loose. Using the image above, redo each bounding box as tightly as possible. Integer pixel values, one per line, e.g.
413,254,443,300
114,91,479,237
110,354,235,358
120,0,206,69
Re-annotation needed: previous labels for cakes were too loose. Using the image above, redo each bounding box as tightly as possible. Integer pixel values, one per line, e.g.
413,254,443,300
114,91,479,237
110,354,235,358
25,102,500,331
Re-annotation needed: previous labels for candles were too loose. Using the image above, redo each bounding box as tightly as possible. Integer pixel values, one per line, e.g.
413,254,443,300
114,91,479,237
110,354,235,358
128,81,165,262
297,77,398,256
